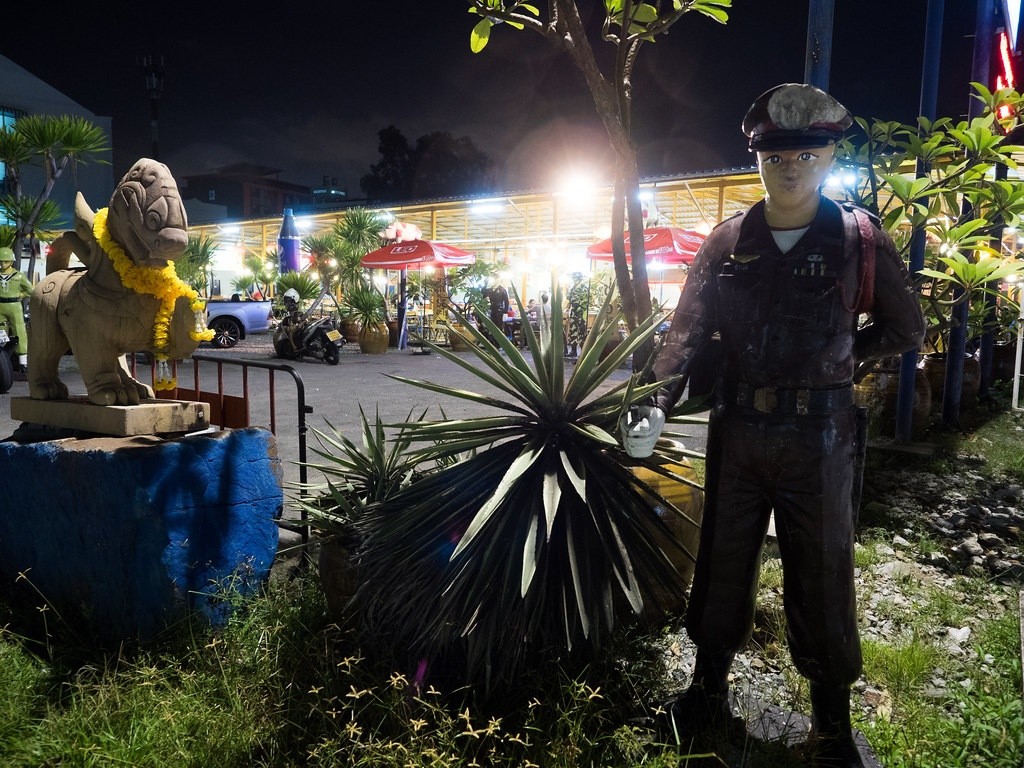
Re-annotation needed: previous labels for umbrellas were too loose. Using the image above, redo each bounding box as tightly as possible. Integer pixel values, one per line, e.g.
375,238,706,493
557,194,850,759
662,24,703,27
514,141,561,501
360,238,477,355
585,226,708,338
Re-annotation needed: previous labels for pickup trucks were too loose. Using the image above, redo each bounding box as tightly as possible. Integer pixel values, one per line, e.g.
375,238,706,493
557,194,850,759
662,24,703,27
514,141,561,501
202,299,274,348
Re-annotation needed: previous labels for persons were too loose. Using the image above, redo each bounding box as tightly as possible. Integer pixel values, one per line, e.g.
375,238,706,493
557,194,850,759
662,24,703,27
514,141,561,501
480,271,509,353
526,293,552,351
565,271,593,359
615,81,927,768
0,246,34,374
525,299,536,318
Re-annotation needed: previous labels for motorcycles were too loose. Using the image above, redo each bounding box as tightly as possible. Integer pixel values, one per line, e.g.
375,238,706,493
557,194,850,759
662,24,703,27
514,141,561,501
0,322,20,394
274,308,346,365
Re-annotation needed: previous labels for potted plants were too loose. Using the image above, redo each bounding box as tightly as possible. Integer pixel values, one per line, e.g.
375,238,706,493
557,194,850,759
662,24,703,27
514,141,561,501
335,293,364,343
417,260,498,352
351,289,389,354
268,407,439,634
835,129,1009,442
918,191,1024,415
